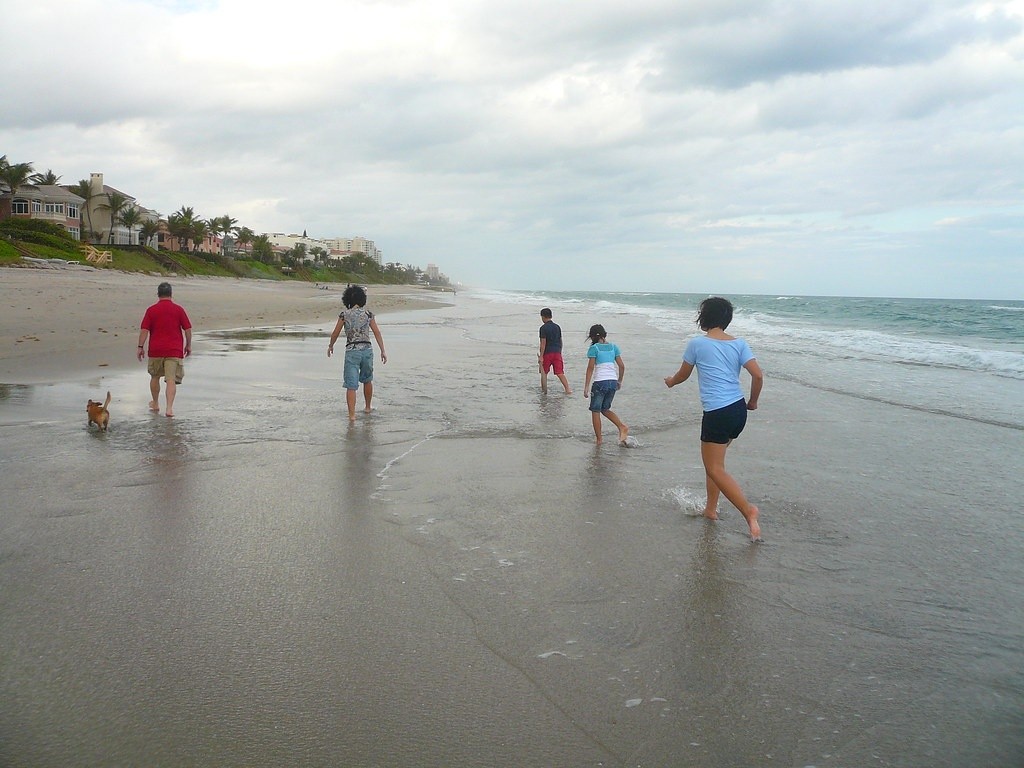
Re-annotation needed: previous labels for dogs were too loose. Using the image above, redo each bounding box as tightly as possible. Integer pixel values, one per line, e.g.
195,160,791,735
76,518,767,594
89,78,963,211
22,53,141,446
85,391,112,432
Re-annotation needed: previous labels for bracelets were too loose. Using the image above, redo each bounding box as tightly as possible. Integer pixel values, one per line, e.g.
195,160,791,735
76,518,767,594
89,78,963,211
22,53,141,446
138,345,143,348
329,344,333,347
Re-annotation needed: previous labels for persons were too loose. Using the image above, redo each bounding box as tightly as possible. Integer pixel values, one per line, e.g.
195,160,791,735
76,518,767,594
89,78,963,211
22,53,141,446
538,308,572,395
136,282,192,417
315,283,367,292
327,284,387,420
442,288,444,294
664,297,764,541
583,324,628,445
